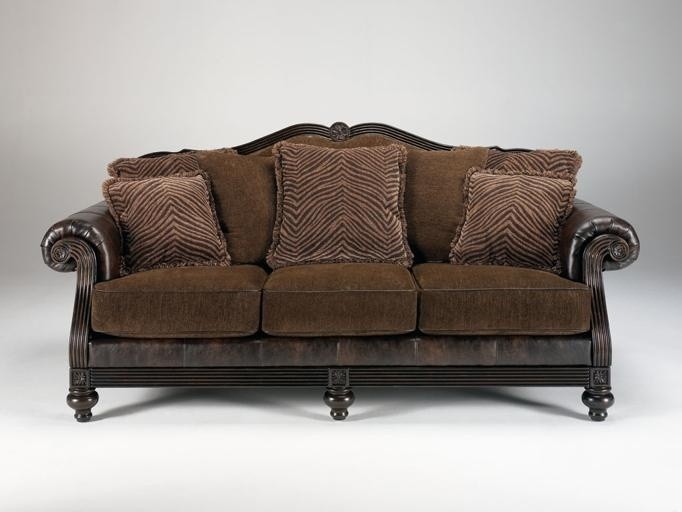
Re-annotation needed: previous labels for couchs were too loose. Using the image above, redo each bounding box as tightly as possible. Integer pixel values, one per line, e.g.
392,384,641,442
41,119,642,423
